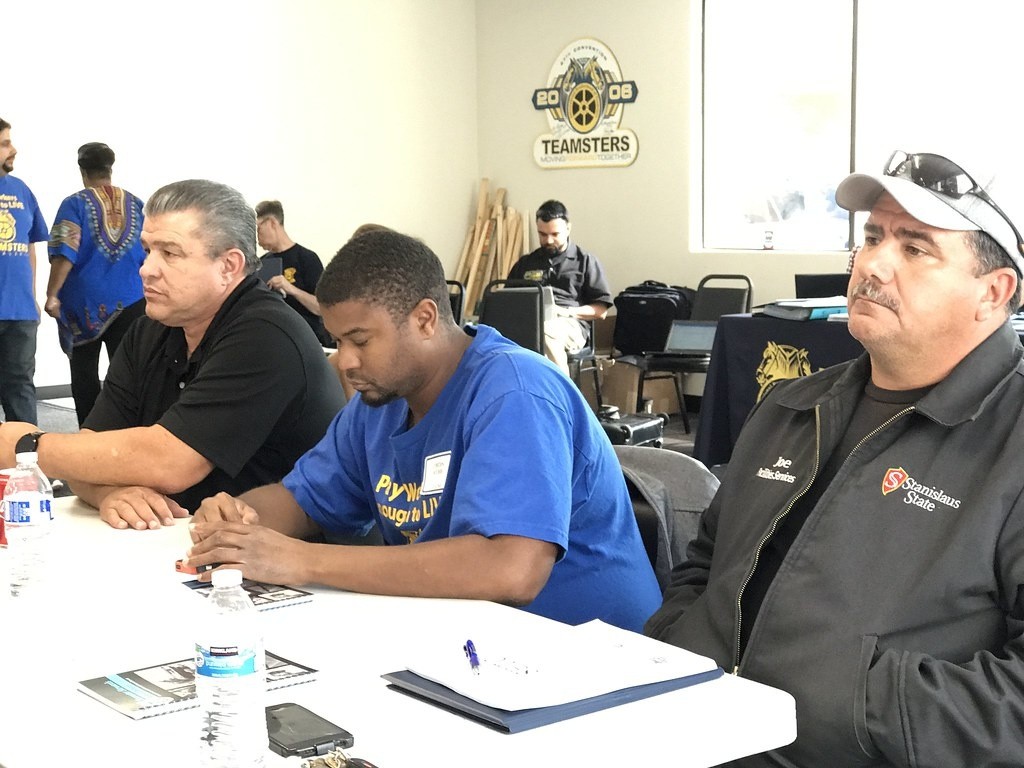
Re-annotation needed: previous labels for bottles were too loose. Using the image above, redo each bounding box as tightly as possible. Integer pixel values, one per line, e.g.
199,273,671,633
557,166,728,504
194,569,269,768
3,451,54,595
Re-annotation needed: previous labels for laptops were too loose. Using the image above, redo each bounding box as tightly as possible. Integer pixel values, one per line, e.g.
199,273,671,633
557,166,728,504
642,320,718,358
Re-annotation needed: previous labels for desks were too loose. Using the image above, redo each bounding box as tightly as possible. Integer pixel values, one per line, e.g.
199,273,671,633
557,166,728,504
691,295,1024,465
0,485,797,768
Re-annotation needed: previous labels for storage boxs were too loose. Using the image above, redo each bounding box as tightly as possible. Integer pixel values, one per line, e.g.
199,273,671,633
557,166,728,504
608,361,679,416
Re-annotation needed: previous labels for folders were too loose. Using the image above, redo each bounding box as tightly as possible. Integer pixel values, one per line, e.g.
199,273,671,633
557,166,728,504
763,294,848,320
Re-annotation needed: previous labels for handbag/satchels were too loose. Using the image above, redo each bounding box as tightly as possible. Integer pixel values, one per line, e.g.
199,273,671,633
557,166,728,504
611,279,692,356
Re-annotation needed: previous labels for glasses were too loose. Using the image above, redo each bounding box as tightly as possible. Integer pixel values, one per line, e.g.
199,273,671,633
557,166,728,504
882,148,1024,260
534,208,569,224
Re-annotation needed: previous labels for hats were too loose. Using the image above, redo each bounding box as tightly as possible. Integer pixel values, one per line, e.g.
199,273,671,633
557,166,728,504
831,146,1024,276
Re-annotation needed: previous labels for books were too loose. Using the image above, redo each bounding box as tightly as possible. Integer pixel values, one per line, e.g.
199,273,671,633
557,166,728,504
76,649,318,721
181,570,314,614
380,618,725,733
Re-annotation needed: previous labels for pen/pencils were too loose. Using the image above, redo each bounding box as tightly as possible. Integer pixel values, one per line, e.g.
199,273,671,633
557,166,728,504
463,640,480,675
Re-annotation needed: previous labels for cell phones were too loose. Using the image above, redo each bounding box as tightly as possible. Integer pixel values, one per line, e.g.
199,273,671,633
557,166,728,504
264,702,354,757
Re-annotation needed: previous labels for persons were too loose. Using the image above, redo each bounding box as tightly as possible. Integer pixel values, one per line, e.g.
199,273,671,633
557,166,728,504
256,200,392,404
642,150,1024,768
504,200,613,378
0,179,347,531
175,231,663,634
0,119,49,427
45,143,145,429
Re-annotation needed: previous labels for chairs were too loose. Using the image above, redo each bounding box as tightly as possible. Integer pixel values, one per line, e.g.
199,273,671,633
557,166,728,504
636,275,756,435
793,274,851,298
476,279,544,352
568,317,605,411
446,280,466,327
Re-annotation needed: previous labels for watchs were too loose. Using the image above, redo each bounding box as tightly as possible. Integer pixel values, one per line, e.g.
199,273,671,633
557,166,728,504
15,431,48,479
